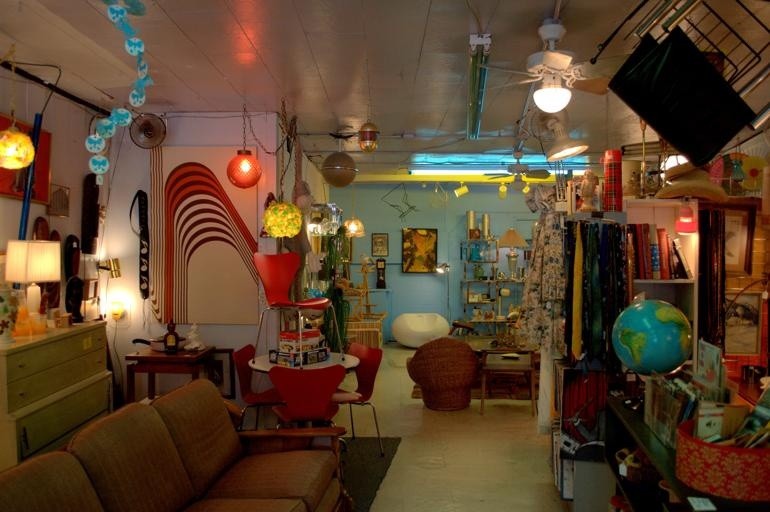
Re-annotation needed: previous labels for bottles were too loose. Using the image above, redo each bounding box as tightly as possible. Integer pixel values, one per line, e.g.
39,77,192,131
473,265,484,280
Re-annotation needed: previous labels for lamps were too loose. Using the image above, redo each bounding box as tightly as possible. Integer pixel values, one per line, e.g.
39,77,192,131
526,110,588,162
533,73,571,113
499,229,527,281
4,232,61,336
129,113,167,148
512,173,526,192
357,162,585,198
227,100,377,257
436,263,451,323
0,43,35,169
97,257,120,279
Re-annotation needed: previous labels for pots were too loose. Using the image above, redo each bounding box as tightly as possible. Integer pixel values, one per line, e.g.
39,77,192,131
131,335,186,353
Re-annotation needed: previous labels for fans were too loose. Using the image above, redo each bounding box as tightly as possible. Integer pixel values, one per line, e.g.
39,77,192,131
483,147,550,180
475,18,631,93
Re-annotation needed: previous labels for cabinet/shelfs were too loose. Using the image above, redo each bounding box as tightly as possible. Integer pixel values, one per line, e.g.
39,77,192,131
548,200,700,511
0,321,114,471
600,367,770,512
460,239,527,335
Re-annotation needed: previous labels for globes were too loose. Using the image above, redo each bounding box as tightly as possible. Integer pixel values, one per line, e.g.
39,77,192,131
612,299,693,413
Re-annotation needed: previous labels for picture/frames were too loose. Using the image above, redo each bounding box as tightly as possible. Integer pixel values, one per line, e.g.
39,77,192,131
0,112,53,205
372,233,389,256
724,203,757,280
723,286,763,358
192,349,236,398
401,228,438,273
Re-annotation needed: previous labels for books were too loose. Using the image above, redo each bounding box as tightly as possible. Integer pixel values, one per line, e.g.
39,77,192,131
627,221,694,279
648,339,770,448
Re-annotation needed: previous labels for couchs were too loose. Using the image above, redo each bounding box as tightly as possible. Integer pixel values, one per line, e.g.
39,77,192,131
0,379,353,512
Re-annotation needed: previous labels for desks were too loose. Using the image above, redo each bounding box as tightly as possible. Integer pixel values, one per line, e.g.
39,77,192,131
467,337,540,385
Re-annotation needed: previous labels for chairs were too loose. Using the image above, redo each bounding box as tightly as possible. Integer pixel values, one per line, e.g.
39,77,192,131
391,313,449,348
406,336,480,411
232,251,384,463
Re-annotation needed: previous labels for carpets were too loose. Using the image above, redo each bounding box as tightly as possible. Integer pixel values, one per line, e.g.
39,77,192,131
340,437,401,512
411,385,539,400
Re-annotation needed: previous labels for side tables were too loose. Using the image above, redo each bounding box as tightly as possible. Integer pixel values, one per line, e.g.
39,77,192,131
126,348,213,404
